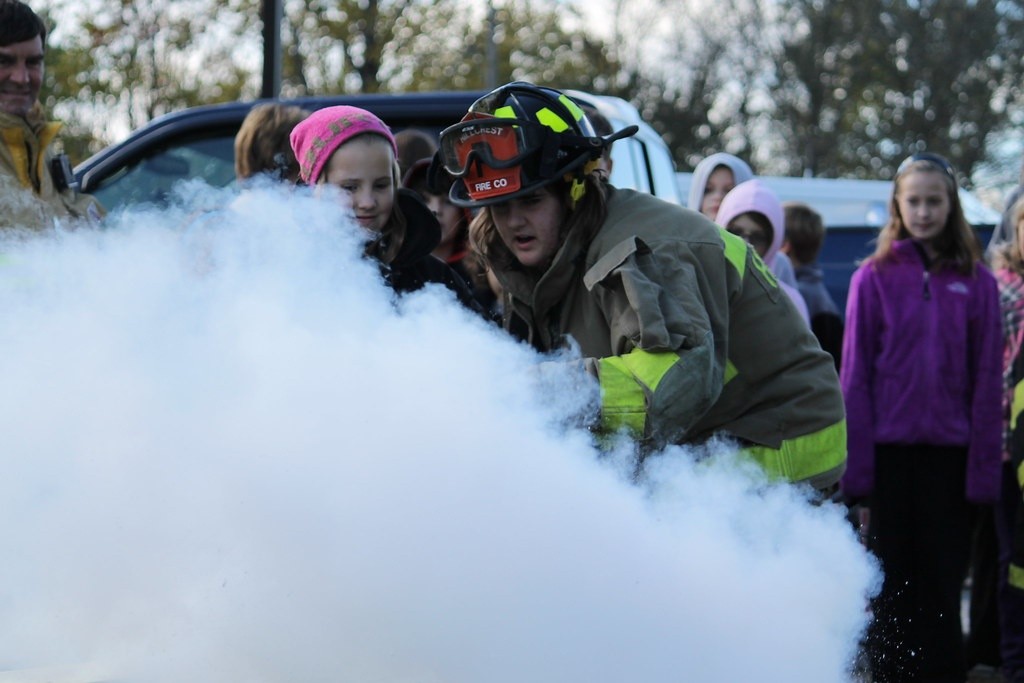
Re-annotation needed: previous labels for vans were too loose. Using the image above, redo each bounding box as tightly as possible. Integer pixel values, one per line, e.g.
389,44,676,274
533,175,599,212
48,87,679,325
674,169,1005,328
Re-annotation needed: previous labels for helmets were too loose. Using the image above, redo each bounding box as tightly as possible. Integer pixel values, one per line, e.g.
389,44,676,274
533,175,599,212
438,82,641,211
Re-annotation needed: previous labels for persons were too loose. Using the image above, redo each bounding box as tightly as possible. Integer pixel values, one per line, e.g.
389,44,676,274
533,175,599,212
841,152,1000,683
685,152,798,290
230,100,614,327
714,180,810,329
0,0,106,229
401,157,496,308
969,185,1024,683
999,349,1024,681
780,201,844,376
437,82,846,506
289,105,486,319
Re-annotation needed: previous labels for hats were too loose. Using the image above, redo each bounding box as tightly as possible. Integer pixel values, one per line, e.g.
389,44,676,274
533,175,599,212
289,106,399,186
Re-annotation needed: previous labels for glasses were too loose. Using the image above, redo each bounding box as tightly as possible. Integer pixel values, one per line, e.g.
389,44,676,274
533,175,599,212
726,224,768,246
439,116,597,177
